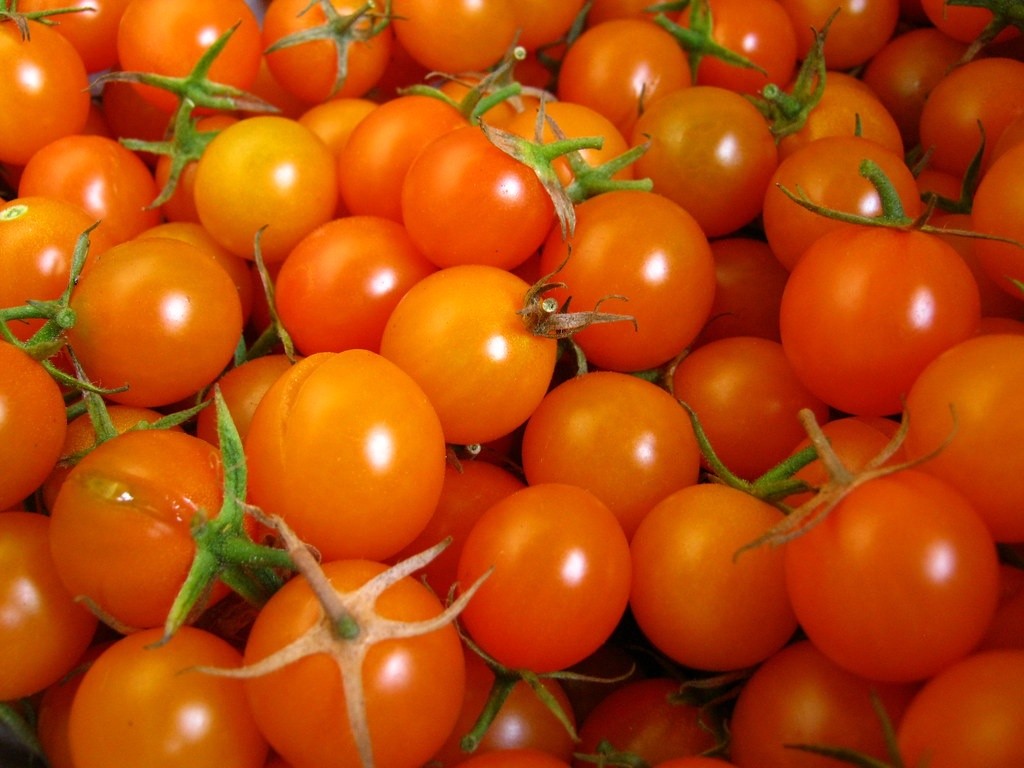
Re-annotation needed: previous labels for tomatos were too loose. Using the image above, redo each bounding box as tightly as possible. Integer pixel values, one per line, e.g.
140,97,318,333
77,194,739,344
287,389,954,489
0,0,1024,768
